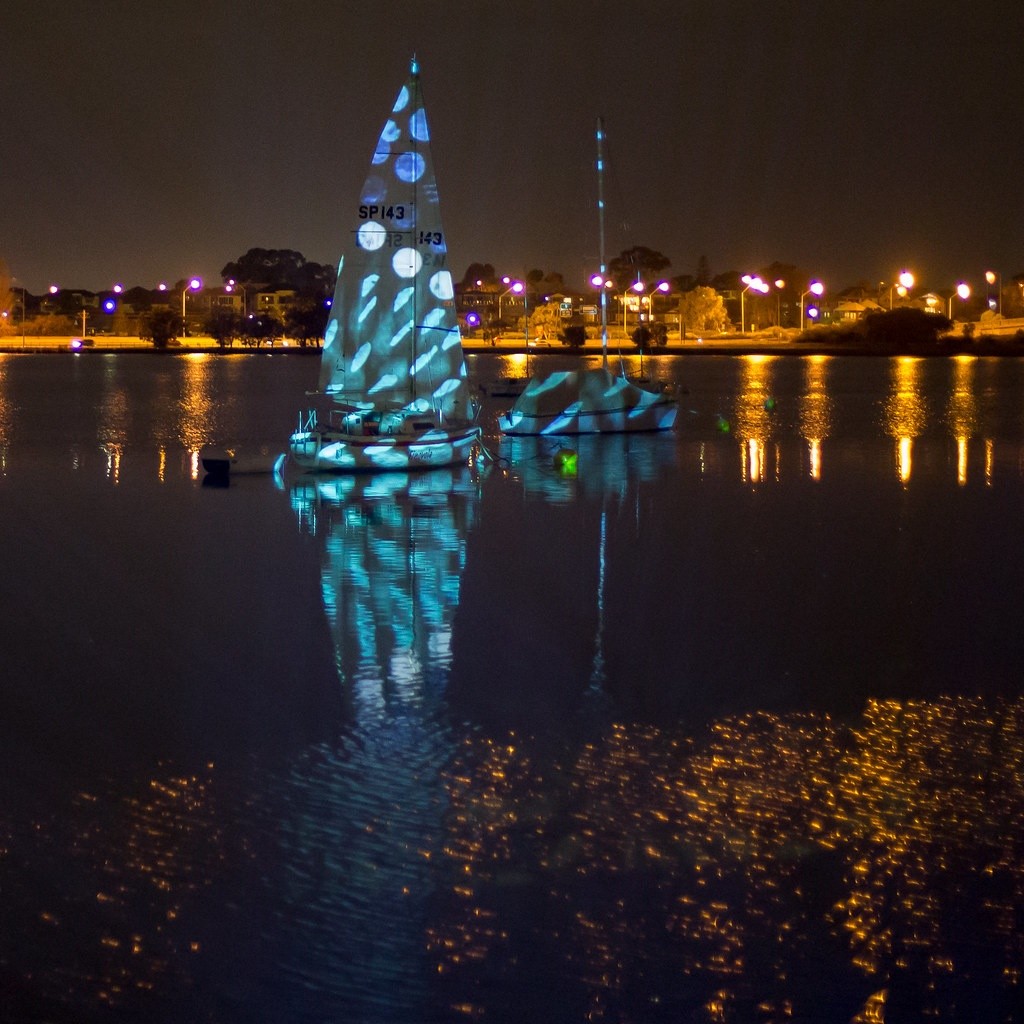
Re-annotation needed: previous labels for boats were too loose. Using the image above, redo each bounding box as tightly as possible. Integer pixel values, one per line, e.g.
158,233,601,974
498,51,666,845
615,347,681,401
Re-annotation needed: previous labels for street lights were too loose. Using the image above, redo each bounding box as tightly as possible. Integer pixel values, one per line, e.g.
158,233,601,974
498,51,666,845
225,279,247,318
648,281,669,333
948,283,970,319
773,277,785,343
502,275,528,327
890,273,914,310
623,280,643,335
182,279,201,339
800,281,823,333
985,271,1003,320
499,283,523,336
739,274,770,337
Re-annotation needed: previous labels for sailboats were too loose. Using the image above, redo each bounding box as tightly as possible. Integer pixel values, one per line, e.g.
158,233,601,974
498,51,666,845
286,53,486,471
490,324,533,396
495,115,683,436
